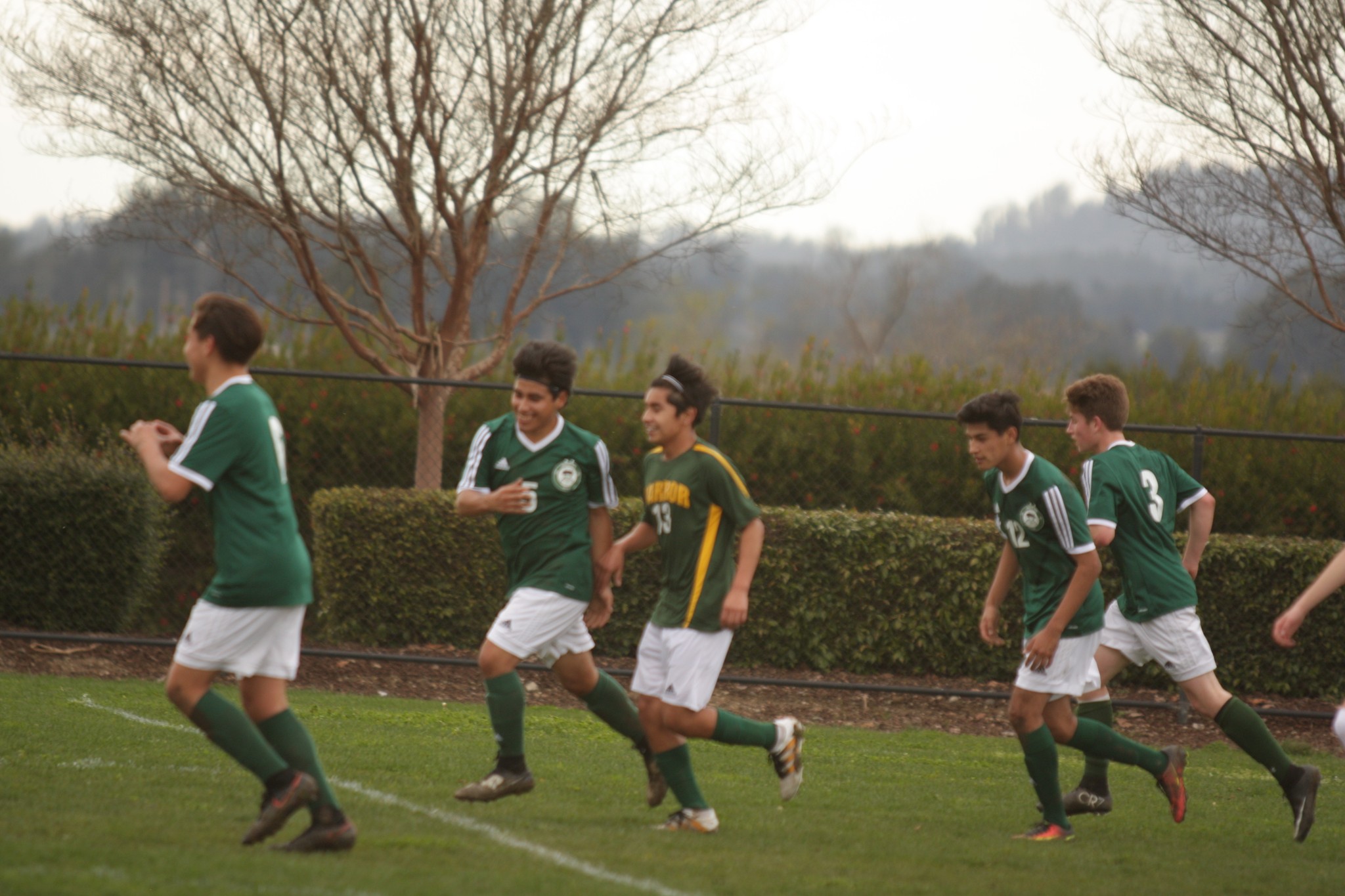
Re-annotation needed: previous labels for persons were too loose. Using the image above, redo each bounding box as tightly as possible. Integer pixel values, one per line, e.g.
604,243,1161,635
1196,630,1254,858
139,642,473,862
957,383,1187,844
1036,372,1319,844
1270,543,1344,742
119,292,363,853
592,351,808,836
451,338,676,811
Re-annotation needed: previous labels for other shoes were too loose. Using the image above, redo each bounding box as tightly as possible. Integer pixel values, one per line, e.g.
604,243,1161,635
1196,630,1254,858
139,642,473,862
270,820,357,854
242,770,318,846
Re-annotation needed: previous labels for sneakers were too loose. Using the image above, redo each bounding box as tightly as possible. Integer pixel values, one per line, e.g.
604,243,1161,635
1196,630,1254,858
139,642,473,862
454,768,533,802
1012,821,1074,842
651,807,719,832
1282,766,1320,842
1153,745,1188,824
645,756,668,808
768,716,805,801
1036,786,1113,816
